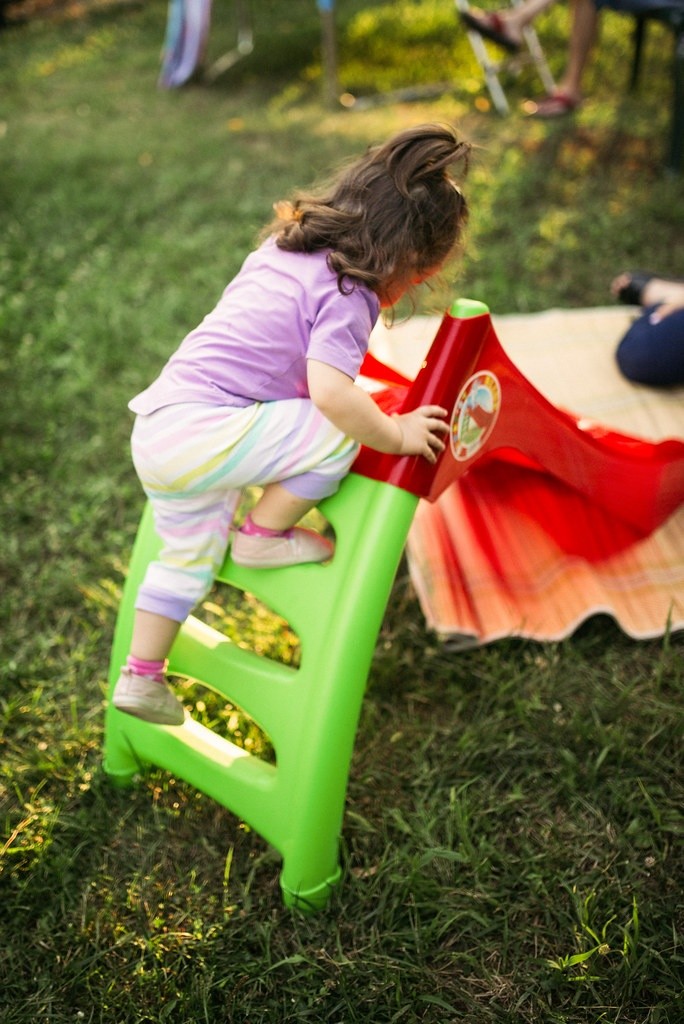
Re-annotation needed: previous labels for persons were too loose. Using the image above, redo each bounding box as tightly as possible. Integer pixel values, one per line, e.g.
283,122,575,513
610,271,684,392
114,125,476,725
452,0,600,118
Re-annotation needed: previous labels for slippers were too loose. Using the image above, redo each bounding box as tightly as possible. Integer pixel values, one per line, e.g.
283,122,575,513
540,96,584,117
460,7,519,53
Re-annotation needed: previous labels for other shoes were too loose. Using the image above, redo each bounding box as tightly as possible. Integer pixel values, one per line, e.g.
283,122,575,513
228,525,334,566
113,666,184,725
